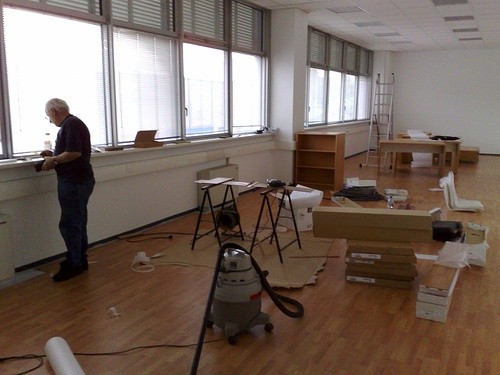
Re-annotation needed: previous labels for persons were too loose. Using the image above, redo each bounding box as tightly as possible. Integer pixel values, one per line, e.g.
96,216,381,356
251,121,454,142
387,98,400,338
38,99,95,281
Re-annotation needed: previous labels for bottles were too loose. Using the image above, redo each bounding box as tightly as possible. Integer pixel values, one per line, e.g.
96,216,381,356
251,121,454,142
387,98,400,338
44,133,52,150
387,196,394,209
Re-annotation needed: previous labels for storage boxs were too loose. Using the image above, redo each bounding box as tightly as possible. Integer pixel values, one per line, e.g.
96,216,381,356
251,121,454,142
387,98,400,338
278,208,313,231
312,207,460,323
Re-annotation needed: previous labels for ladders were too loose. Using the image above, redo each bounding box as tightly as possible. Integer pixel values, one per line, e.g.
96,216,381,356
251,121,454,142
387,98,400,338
366,73,394,166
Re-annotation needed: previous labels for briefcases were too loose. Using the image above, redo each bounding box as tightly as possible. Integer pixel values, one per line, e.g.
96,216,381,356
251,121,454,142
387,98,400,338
432,219,463,240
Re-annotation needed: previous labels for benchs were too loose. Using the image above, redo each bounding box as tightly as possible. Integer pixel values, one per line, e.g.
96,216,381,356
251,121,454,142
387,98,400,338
431,146,480,166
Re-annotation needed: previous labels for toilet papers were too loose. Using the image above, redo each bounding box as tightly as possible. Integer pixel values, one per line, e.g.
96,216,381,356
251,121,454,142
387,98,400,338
44,337,85,375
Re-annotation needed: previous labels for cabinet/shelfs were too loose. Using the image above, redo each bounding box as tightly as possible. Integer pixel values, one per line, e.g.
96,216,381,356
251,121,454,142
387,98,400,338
296,130,345,199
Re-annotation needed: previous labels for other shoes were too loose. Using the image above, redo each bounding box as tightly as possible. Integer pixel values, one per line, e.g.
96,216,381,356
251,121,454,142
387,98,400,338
82,260,88,270
52,264,82,283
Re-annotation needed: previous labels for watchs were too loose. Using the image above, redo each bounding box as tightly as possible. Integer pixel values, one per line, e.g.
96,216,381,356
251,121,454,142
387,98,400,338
53,156,58,165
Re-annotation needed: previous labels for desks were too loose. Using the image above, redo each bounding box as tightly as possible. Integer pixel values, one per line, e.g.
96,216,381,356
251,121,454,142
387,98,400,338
380,133,463,178
